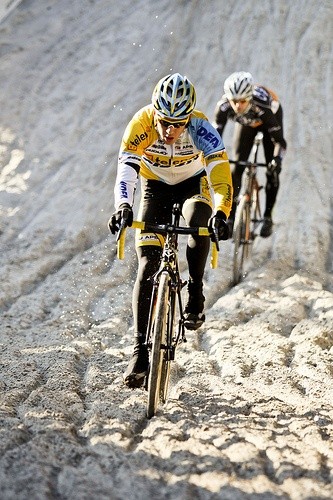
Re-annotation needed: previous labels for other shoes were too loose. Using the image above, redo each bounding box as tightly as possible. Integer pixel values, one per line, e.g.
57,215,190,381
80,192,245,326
182,298,206,331
224,219,234,236
259,220,273,238
123,353,151,388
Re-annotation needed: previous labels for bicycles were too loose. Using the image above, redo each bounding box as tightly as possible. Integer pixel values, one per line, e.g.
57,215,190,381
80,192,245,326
115,198,224,419
229,137,275,283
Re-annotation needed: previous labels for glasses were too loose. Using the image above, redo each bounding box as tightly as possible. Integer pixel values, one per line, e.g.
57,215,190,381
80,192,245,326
157,117,190,128
231,100,250,104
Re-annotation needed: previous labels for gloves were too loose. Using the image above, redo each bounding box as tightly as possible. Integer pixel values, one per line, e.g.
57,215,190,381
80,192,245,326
207,211,231,242
106,203,134,235
268,157,282,176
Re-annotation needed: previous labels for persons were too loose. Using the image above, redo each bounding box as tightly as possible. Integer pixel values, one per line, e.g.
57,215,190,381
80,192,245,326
212,71,286,239
106,73,233,388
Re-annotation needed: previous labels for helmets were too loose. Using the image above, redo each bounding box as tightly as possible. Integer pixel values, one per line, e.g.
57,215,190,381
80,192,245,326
223,71,254,100
152,73,196,119
